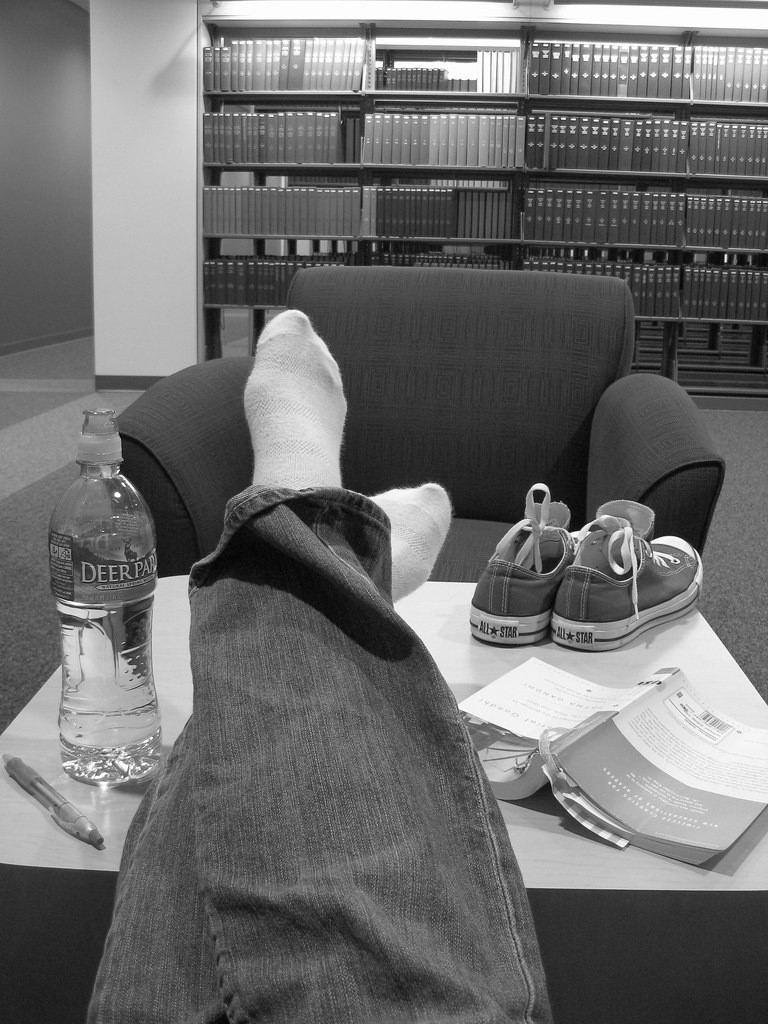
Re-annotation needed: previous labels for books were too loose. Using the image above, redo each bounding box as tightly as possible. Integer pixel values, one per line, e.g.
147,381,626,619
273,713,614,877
457,656,768,865
201,39,768,370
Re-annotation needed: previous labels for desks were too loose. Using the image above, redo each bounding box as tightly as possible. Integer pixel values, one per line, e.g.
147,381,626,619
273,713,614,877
0,573,768,1023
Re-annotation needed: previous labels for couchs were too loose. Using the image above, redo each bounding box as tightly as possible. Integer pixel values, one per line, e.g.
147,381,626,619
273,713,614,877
112,263,727,584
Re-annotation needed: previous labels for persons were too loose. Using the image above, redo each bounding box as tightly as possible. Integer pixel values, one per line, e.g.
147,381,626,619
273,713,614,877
86,309,564,1024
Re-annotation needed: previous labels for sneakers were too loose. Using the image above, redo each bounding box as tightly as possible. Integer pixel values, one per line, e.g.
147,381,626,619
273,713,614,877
470,485,597,646
551,498,705,652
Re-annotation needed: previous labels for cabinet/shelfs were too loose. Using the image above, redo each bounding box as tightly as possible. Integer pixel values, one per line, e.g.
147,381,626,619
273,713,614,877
194,0,768,411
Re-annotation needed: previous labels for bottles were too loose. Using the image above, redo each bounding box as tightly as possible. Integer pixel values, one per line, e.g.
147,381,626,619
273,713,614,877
48,409,163,787
